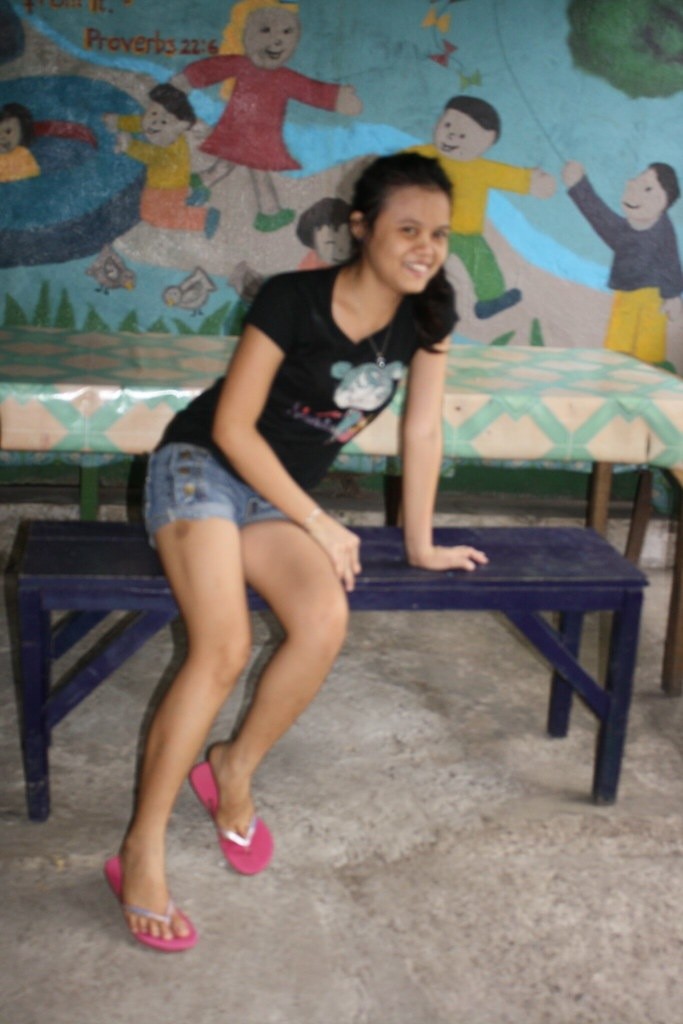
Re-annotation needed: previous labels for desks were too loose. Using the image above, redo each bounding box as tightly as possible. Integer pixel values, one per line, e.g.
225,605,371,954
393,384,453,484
0,325,683,699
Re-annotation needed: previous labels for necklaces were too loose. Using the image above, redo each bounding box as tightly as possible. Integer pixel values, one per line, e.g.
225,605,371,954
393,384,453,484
361,315,393,368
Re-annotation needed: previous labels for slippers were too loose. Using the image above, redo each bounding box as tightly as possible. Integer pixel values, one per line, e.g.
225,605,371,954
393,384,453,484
188,761,272,875
103,855,198,950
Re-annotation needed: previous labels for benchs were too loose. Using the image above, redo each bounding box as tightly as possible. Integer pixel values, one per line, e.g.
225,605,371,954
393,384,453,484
7,518,650,821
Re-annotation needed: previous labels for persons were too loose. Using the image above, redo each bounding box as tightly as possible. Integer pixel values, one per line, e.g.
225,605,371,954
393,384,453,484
103,151,489,952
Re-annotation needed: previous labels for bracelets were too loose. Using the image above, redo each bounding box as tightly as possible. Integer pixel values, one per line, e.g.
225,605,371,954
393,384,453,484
302,508,322,527
